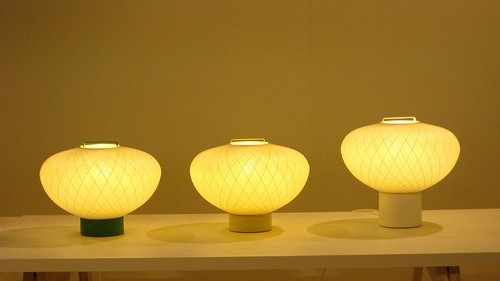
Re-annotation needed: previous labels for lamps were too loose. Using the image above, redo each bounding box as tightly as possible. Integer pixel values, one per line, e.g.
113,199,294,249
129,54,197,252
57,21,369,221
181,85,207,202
39,140,163,238
189,137,311,235
339,117,461,231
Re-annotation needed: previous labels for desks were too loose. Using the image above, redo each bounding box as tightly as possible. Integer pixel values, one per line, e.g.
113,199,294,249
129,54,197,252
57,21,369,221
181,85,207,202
2,207,500,281
